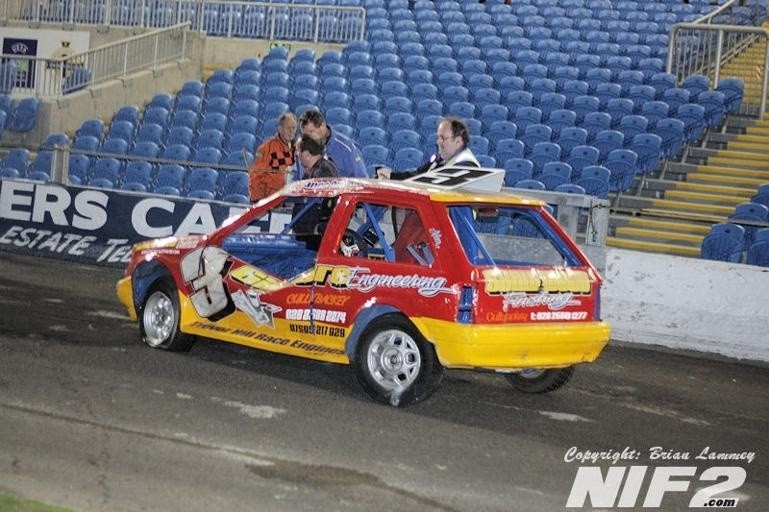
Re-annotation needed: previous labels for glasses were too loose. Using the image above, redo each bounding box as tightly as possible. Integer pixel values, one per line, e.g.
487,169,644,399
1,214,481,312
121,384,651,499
438,136,456,143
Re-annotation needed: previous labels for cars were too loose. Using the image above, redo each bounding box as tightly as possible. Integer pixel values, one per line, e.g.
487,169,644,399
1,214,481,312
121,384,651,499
114,175,613,408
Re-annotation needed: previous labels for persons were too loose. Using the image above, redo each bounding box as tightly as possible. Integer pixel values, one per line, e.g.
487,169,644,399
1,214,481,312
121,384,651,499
293,110,370,232
290,131,338,251
246,111,300,205
376,118,483,181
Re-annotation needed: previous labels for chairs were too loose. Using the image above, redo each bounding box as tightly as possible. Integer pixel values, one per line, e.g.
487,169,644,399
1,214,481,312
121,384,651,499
0,0,769,268
